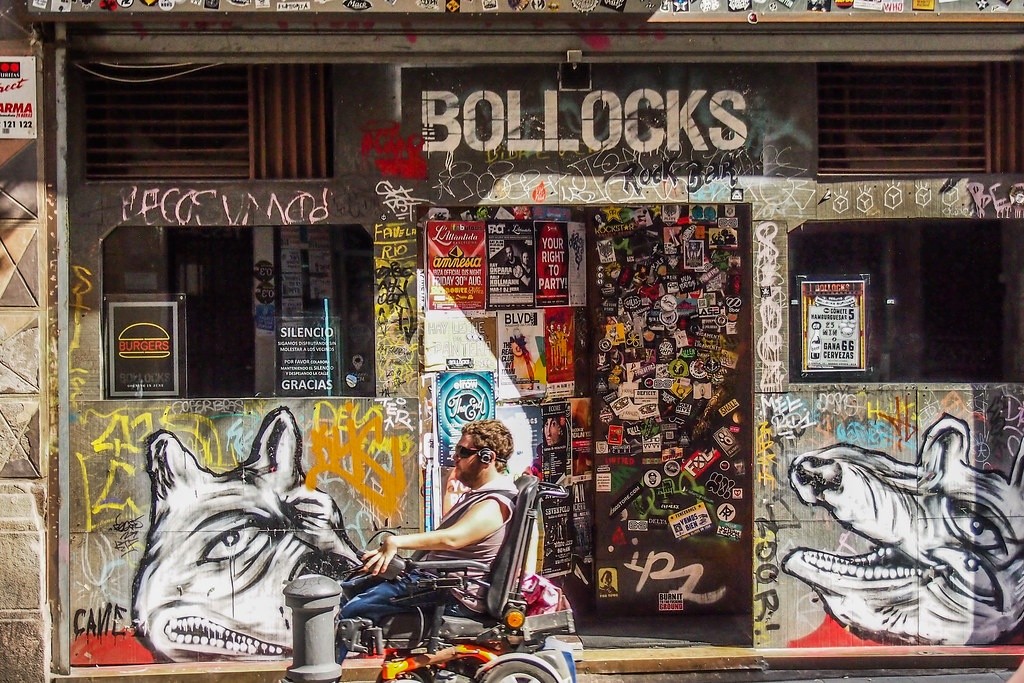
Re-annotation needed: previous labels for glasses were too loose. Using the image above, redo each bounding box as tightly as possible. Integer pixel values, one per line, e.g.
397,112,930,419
454,443,479,457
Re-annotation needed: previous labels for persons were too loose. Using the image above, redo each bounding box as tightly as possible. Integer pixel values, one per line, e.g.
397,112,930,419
501,244,521,278
518,249,533,292
441,468,473,517
542,413,566,450
277,419,520,683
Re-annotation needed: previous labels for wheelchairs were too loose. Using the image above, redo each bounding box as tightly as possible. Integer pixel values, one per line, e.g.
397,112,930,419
338,475,576,683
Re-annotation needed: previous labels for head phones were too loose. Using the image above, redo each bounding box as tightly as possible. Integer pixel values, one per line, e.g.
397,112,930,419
477,447,507,464
